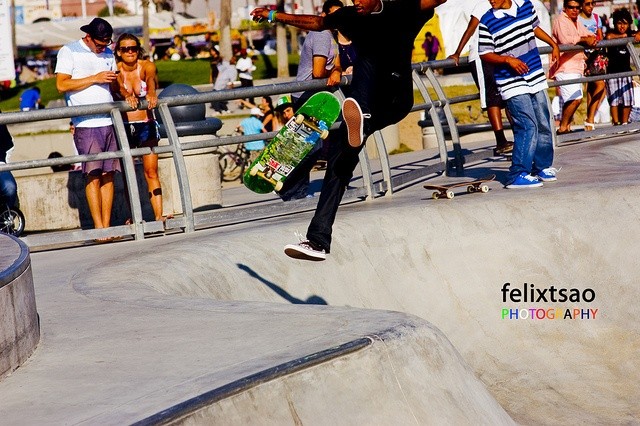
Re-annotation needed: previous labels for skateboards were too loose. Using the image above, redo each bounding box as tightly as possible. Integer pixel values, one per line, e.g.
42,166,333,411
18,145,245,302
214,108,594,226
243,91,341,194
500,152,512,161
423,174,496,199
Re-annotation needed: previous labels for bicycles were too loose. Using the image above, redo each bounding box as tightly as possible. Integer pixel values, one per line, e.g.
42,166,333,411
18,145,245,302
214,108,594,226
218,128,272,183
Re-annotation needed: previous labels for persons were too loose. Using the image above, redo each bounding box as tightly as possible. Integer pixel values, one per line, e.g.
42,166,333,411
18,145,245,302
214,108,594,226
17,87,44,111
54,17,124,244
550,0,598,135
604,8,640,126
336,32,354,74
250,0,448,262
208,44,253,107
578,0,606,131
111,33,175,225
290,0,343,198
421,31,441,60
448,0,514,155
477,0,561,189
236,97,293,163
47,119,83,171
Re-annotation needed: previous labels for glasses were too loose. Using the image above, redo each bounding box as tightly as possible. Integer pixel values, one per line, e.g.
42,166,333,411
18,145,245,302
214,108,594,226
584,1,597,7
119,46,138,53
90,38,113,49
566,5,580,9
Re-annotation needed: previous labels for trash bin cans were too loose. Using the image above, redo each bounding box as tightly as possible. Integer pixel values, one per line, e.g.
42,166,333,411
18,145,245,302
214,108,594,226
157,85,222,216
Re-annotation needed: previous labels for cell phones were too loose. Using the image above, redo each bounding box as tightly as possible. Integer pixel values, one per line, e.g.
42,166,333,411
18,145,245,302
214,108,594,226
115,71,120,74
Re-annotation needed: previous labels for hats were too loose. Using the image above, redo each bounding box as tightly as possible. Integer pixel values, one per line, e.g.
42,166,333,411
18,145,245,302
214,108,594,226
80,18,113,40
275,97,292,109
249,106,265,116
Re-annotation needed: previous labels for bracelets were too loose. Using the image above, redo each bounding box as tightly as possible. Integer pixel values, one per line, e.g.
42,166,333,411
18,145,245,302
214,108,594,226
331,66,342,74
267,10,277,24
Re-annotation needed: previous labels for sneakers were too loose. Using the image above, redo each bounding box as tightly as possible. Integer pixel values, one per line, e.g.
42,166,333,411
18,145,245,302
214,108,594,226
342,98,364,147
507,172,543,188
284,241,326,262
537,168,558,183
